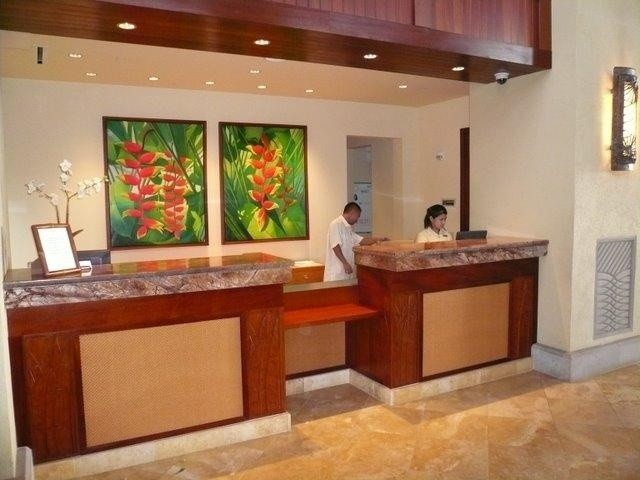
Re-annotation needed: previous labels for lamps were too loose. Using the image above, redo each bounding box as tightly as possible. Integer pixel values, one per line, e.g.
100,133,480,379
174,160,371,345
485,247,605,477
611,65,639,174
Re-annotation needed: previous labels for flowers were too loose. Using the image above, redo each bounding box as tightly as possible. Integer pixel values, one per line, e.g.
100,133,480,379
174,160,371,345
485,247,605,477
24,153,110,237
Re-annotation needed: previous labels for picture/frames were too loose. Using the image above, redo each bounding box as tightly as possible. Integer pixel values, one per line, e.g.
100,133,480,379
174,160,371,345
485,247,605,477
219,121,310,247
31,221,82,277
101,117,210,250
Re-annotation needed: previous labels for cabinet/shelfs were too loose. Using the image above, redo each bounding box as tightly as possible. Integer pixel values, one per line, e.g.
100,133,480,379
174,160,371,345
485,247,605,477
285,259,324,286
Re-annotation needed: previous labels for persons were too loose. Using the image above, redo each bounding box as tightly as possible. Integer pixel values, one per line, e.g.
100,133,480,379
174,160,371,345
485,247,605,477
322,202,391,284
414,204,453,243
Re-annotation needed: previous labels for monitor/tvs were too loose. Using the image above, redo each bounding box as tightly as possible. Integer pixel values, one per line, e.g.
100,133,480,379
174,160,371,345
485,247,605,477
455,230,487,239
77,250,111,265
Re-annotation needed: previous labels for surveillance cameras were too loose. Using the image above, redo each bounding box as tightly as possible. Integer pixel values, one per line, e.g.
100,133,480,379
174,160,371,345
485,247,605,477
495,72,509,84
